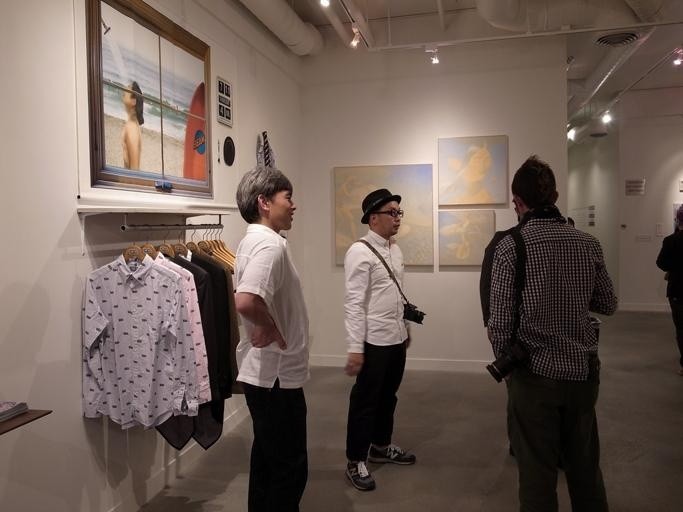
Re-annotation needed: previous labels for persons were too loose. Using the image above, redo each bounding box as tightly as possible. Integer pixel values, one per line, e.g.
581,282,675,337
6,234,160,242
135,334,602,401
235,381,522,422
121,83,146,175
233,170,311,512
654,202,682,376
479,156,616,512
341,187,420,496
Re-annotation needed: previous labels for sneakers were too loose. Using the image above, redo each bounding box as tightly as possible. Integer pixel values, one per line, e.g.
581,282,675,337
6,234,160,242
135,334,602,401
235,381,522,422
345,458,376,491
368,444,416,465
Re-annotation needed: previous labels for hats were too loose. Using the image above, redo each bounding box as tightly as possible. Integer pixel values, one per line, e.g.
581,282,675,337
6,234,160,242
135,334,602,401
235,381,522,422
361,189,401,224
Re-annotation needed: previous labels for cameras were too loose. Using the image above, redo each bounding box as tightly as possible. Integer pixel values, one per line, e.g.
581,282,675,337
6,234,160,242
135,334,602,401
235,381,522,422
485,338,530,384
403,302,427,325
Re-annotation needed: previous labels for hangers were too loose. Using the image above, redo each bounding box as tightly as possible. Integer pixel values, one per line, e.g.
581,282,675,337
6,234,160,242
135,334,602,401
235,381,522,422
123,224,236,274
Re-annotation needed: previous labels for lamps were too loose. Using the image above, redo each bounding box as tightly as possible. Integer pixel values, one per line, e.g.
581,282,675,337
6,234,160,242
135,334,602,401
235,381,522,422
431,48,440,64
673,54,683,65
601,110,611,124
350,23,360,49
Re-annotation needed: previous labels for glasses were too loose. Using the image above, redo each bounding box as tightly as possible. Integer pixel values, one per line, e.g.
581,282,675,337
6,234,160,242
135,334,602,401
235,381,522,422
370,209,404,218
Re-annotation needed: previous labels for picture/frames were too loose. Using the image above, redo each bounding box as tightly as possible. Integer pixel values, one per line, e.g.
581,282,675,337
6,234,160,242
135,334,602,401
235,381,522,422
84,0,214,199
215,75,233,128
